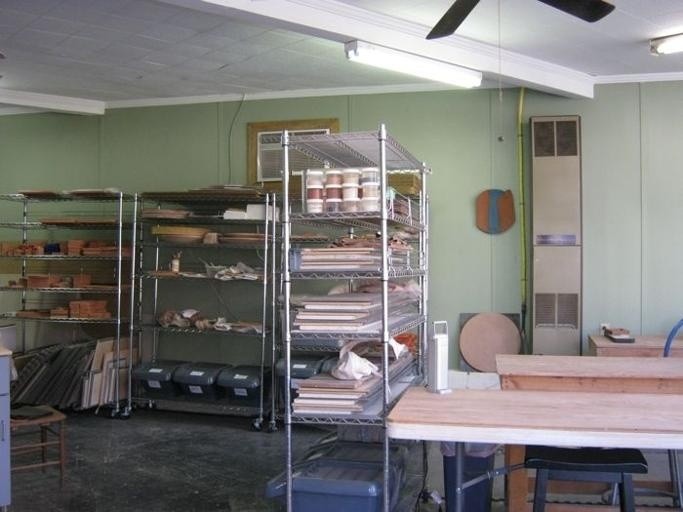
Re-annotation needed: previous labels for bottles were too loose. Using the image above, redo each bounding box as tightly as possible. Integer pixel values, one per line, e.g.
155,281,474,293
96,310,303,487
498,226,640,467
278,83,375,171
305,170,381,213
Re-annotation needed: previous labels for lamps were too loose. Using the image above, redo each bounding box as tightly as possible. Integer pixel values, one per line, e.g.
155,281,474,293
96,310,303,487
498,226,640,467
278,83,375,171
648,32,683,56
343,40,483,89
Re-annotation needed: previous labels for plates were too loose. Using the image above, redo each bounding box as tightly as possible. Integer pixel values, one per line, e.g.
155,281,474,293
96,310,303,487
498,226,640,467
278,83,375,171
218,232,275,243
151,226,208,242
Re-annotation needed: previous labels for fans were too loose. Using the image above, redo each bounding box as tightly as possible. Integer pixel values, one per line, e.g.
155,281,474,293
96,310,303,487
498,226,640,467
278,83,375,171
426,0,616,39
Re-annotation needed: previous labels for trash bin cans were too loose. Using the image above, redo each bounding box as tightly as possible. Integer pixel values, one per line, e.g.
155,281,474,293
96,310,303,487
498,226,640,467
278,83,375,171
441,441,497,512
